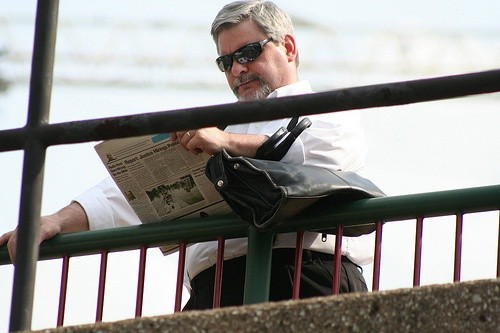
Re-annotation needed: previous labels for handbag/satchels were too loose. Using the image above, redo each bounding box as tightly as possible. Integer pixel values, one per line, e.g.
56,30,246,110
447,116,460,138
205,117,387,242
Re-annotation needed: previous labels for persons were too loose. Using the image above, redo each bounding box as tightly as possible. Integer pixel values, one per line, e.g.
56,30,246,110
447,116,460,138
1,1,368,312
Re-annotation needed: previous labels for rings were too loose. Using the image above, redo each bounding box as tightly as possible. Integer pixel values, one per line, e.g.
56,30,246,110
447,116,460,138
187,131,192,139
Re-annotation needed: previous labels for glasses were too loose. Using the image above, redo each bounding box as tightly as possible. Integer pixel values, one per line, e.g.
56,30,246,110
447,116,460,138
216,37,272,73
